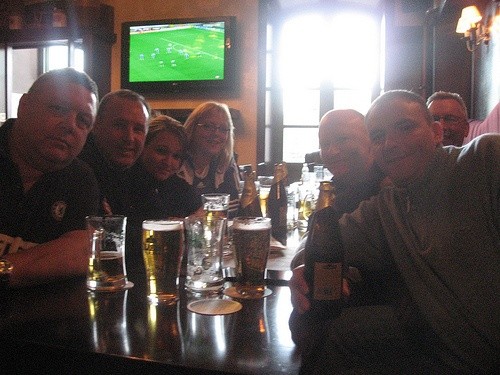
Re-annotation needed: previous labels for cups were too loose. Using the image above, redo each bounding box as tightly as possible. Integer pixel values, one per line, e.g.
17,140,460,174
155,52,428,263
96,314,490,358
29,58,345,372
52,9,67,27
228,297,271,368
233,217,272,296
141,219,185,301
145,298,183,363
87,290,129,356
314,165,332,182
286,180,319,233
201,192,230,255
257,176,274,211
183,291,227,364
8,16,24,29
238,181,261,200
184,216,226,294
85,215,128,291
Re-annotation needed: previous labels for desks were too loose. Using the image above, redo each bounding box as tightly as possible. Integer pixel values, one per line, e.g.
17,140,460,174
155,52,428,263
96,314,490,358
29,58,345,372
0,169,336,375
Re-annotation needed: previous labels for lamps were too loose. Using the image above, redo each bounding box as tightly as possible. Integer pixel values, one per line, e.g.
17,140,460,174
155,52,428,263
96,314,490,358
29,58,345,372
456,5,492,52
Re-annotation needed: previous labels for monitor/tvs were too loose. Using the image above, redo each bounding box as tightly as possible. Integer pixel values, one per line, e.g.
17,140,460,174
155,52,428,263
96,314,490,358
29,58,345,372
120,14,237,90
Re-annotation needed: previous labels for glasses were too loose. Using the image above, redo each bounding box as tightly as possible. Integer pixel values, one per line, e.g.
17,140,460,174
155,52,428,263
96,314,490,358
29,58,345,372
196,121,230,134
432,114,467,125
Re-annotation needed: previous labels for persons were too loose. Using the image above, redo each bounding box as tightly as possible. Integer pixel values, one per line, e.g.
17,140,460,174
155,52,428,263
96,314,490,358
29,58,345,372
77,88,240,254
0,68,101,289
137,43,191,68
289,90,500,375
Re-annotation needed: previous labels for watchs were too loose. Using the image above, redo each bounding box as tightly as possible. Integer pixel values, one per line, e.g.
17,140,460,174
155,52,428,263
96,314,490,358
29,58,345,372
0,258,14,289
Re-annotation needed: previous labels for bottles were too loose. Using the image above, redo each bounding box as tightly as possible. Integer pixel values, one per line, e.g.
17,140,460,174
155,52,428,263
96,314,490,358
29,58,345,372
267,163,287,246
303,181,343,320
237,166,263,217
301,163,309,179
